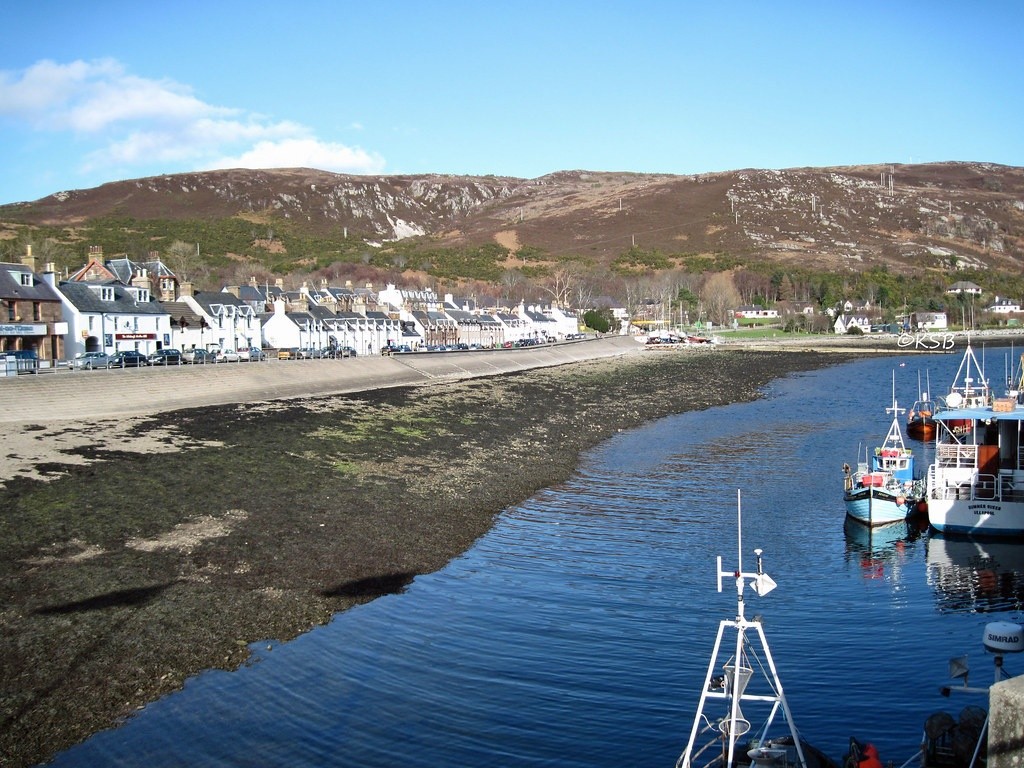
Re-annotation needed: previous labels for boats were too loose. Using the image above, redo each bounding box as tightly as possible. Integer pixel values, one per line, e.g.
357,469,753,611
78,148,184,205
838,331,1024,535
843,513,912,557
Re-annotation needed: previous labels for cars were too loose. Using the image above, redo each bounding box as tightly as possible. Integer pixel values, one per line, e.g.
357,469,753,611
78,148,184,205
108,350,147,367
278,346,357,360
504,333,587,349
211,348,242,363
181,348,216,363
397,344,412,353
67,351,118,369
417,342,483,353
0,348,40,373
381,345,399,356
237,346,266,361
145,349,184,366
